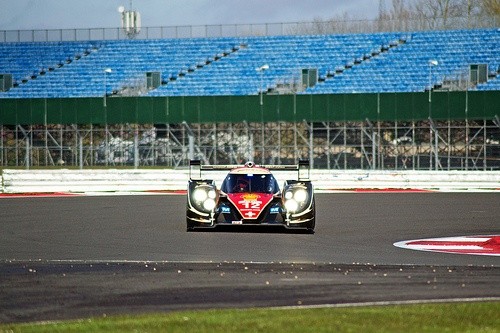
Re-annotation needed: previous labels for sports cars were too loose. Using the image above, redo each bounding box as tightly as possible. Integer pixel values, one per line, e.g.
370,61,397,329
186,158,316,233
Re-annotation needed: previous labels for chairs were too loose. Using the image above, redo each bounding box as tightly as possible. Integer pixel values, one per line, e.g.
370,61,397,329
2,34,500,101
